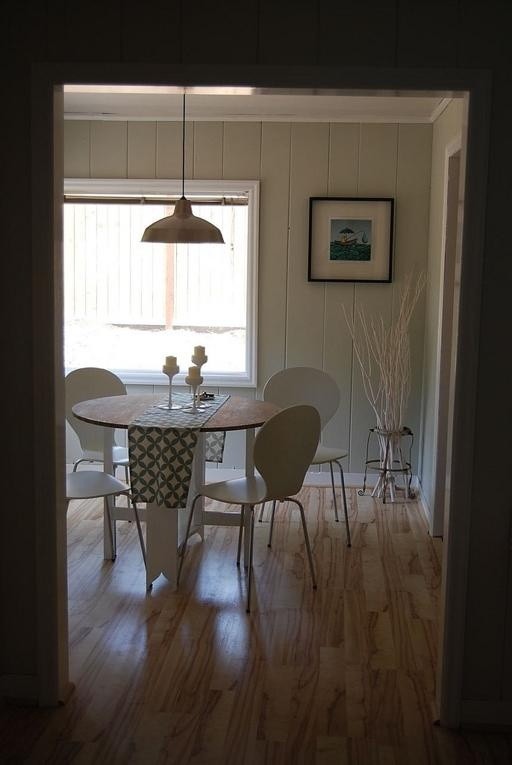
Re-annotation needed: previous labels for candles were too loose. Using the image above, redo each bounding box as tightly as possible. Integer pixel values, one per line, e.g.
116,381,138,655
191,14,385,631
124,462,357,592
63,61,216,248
188,367,201,380
166,355,177,371
194,345,205,360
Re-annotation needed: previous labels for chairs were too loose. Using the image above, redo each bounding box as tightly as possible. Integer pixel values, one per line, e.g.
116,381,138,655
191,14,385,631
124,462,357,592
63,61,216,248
67,368,133,523
175,405,321,616
257,366,351,550
68,471,153,589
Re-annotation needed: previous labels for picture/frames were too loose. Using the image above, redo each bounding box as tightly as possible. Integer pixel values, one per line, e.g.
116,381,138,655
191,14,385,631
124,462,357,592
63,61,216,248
307,196,394,283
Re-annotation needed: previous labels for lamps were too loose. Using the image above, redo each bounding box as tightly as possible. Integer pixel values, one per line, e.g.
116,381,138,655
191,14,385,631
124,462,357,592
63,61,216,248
139,94,224,243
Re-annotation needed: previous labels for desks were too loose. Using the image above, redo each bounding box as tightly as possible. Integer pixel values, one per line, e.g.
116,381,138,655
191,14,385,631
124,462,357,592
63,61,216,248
72,393,284,591
